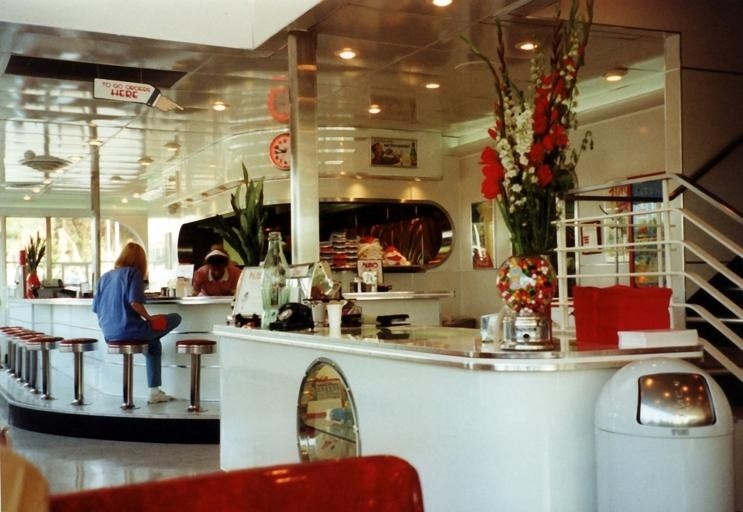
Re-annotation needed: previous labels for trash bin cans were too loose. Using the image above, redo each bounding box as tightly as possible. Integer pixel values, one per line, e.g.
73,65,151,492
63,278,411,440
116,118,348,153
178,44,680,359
590,353,740,511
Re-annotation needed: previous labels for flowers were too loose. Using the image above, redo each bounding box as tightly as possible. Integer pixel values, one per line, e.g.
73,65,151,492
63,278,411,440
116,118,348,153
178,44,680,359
467,2,596,257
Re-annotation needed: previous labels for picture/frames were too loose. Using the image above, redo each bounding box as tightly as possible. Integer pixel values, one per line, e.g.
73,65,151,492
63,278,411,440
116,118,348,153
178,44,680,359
229,266,264,318
469,197,495,270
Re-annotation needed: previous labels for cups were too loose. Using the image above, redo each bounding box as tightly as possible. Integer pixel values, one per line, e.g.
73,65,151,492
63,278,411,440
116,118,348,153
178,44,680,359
326,302,344,338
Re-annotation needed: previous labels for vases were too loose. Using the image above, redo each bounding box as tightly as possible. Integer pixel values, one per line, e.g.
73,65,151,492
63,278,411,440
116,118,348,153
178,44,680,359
495,254,558,311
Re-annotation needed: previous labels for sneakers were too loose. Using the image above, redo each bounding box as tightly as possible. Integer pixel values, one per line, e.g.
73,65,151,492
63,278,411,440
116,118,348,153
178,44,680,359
146,390,175,404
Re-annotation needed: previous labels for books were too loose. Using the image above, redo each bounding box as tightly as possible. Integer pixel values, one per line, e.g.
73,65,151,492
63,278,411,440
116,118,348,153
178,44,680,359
617,330,698,350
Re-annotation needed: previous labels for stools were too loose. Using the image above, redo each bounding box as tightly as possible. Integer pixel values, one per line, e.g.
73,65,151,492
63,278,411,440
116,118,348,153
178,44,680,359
0,324,216,411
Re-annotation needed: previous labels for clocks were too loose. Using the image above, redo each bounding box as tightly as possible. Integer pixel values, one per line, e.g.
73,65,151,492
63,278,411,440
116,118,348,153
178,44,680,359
269,131,291,170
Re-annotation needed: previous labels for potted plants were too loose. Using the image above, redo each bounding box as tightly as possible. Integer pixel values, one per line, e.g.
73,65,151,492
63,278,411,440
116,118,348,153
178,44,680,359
24,232,46,300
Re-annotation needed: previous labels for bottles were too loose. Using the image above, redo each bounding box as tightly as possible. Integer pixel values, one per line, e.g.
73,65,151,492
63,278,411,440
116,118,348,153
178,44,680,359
261,232,293,332
410,143,417,166
176,277,185,297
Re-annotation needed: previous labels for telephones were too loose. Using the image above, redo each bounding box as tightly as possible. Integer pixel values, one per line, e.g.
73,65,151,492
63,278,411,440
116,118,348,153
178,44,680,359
269,303,315,332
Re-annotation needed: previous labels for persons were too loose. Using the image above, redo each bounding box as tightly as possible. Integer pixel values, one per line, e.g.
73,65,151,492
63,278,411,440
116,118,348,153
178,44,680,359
94,77,184,112
189,245,242,296
371,142,400,164
93,243,182,403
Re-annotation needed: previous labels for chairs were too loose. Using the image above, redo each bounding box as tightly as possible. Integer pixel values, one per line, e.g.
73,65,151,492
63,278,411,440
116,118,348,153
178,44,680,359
571,283,672,352
